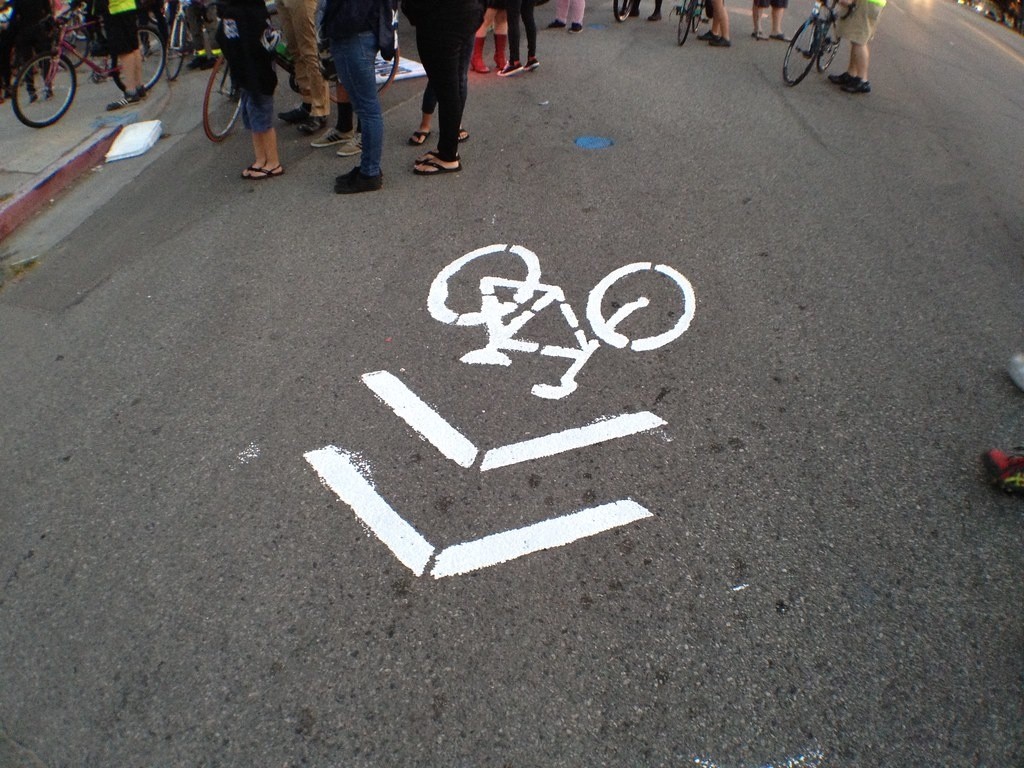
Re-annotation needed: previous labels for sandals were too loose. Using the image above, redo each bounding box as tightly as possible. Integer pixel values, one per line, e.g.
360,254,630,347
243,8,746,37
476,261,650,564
242,164,286,180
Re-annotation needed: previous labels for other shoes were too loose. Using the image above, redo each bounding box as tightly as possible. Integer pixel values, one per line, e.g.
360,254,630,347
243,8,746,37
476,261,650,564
28,45,218,112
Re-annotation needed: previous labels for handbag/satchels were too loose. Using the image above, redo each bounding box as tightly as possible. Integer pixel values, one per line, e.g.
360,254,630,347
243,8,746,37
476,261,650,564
380,0,399,61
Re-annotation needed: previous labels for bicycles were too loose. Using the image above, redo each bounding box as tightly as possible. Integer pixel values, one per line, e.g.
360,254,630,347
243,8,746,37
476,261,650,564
0,0,222,129
676,0,710,47
613,0,638,23
782,0,856,86
203,0,401,142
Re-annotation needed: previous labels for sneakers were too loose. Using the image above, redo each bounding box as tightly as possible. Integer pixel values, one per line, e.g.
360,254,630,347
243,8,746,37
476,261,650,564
546,19,583,34
981,448,1024,500
841,78,871,93
310,126,362,156
336,166,362,184
334,172,382,193
497,61,523,76
828,73,853,86
523,57,539,71
277,108,329,137
698,30,731,47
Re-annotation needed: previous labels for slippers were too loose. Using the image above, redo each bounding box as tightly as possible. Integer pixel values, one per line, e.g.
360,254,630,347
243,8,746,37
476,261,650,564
770,32,793,42
458,128,469,142
413,149,462,176
751,30,769,40
408,131,430,146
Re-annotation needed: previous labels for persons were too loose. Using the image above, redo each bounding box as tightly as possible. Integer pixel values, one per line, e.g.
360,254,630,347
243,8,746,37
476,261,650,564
827,0,887,94
548,0,585,33
139,0,180,56
472,0,549,75
216,0,284,179
183,0,219,71
71,0,148,111
274,0,330,135
0,0,53,105
753,0,792,42
630,0,663,21
697,0,731,47
311,0,394,193
401,0,486,176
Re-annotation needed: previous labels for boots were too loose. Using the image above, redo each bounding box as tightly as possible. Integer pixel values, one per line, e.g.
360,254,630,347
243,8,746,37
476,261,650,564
647,0,663,22
471,37,491,73
628,0,642,18
493,34,506,68
